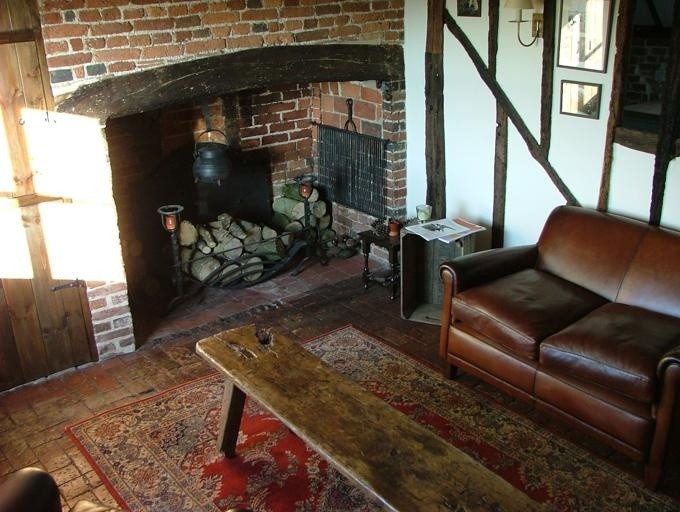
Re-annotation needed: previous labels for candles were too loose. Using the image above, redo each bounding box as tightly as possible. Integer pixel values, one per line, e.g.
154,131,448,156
166,215,177,229
301,186,310,197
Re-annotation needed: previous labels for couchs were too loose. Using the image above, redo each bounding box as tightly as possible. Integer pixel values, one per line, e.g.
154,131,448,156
436,203,678,492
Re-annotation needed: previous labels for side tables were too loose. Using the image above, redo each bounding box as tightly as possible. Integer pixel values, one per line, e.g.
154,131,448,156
356,224,418,305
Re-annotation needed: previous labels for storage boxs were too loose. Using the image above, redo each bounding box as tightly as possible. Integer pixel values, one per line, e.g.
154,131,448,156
400,219,476,326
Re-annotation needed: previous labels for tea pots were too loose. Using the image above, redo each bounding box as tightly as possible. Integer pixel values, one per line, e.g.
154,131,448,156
192,129,230,186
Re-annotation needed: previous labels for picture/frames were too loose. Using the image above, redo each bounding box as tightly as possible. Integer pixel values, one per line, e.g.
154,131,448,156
457,1,481,17
560,80,602,120
556,0,615,73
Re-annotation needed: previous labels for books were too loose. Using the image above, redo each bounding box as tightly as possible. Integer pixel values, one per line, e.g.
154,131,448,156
405,218,482,243
439,227,487,244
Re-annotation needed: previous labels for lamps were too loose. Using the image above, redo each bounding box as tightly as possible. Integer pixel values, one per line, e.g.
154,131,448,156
503,1,543,47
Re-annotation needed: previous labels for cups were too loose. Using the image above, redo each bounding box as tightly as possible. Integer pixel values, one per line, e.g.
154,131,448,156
415,205,433,222
388,221,400,233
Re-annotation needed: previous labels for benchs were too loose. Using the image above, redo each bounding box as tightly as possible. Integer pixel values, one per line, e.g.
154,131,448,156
194,323,547,511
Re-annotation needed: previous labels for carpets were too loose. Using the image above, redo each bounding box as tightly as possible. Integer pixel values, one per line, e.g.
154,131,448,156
60,323,679,512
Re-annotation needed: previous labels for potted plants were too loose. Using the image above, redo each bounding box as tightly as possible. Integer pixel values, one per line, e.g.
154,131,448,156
369,212,418,241
